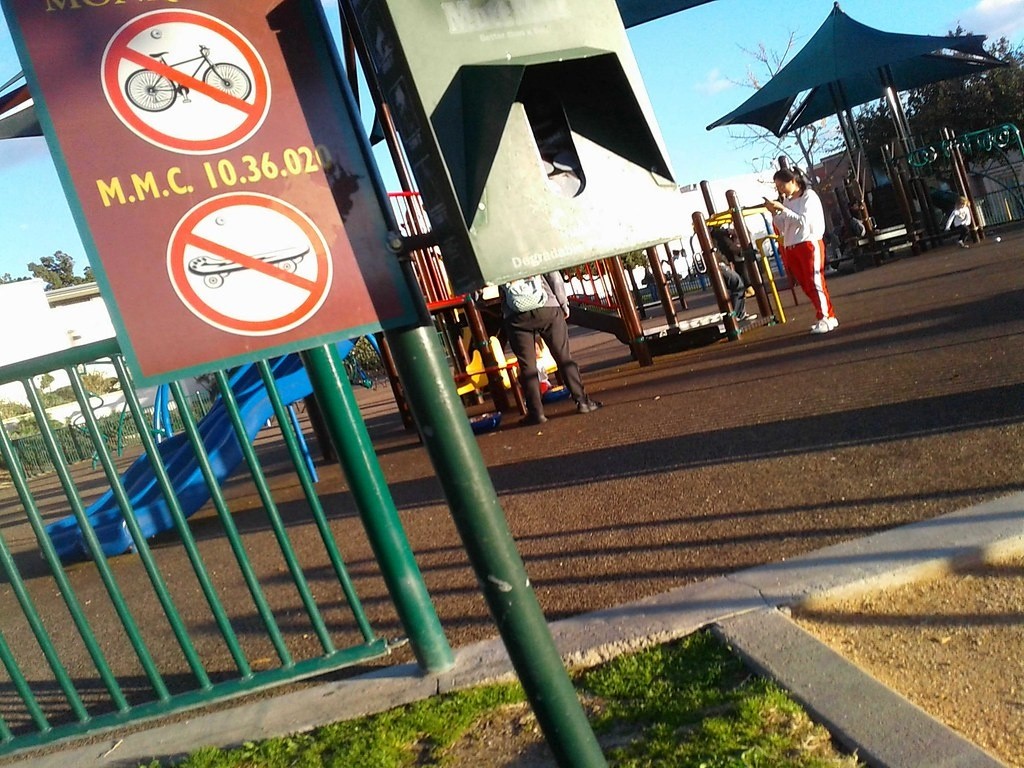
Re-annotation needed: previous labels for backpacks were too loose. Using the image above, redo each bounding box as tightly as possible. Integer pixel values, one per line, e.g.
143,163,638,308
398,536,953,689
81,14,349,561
501,275,549,312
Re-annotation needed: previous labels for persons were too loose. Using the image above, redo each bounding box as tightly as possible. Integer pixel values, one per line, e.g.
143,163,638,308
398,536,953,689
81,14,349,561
707,224,758,322
945,197,971,247
765,167,839,333
498,271,601,424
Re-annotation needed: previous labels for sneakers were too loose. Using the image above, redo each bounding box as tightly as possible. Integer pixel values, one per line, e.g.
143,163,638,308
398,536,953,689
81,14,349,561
811,317,838,333
519,414,547,425
578,400,603,413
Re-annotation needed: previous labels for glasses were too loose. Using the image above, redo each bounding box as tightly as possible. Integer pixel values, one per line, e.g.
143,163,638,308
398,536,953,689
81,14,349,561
774,181,787,192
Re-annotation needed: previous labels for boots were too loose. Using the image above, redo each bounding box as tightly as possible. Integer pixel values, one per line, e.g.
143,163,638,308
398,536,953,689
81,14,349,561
745,286,755,298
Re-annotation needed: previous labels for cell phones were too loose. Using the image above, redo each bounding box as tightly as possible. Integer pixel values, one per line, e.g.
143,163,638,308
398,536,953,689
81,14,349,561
762,196,771,202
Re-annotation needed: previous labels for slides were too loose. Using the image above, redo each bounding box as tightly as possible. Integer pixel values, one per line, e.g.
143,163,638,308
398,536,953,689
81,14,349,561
33,334,359,563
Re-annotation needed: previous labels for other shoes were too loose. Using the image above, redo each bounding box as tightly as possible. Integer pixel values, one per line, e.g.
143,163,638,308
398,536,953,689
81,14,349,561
964,245,969,248
737,314,758,322
959,240,964,247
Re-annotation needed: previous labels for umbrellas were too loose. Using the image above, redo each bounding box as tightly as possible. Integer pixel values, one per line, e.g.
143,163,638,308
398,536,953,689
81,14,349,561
706,2,1008,139
